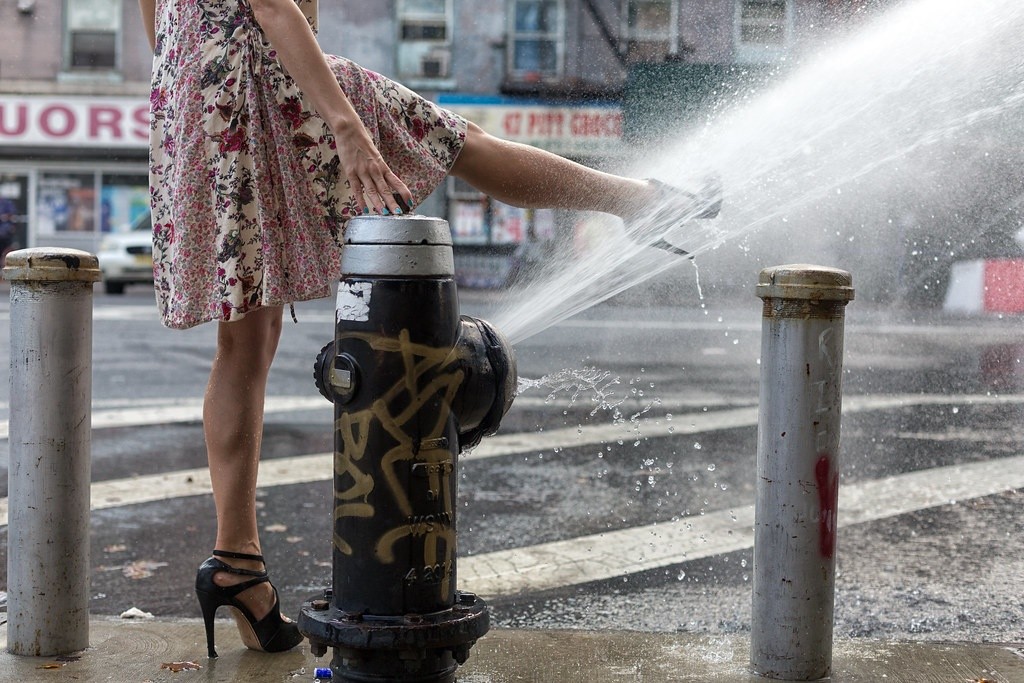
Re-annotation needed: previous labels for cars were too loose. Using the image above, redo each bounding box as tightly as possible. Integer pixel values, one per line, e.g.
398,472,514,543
95,231,159,295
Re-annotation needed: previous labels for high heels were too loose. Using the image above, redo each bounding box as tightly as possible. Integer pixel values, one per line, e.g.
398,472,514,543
624,168,722,260
195,549,304,657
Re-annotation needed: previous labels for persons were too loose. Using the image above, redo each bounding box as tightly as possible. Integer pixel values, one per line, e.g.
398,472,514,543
137,0,722,658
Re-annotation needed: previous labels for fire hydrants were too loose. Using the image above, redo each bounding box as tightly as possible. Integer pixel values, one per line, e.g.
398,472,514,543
295,216,521,683
